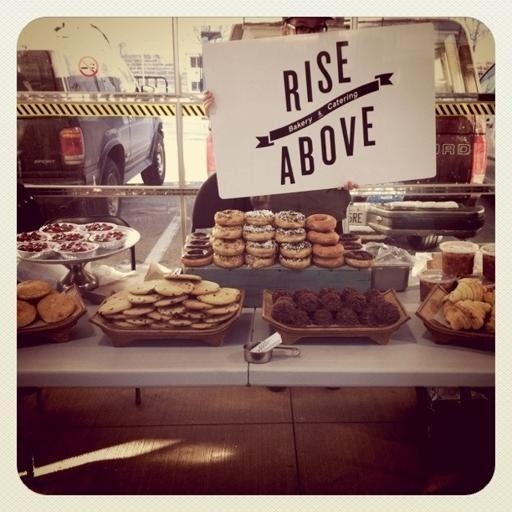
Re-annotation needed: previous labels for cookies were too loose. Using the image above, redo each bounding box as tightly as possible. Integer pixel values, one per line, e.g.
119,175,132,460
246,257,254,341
17,276,77,328
97,272,242,329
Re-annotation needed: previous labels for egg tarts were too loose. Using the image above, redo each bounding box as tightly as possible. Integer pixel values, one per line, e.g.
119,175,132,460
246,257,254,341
17,220,127,260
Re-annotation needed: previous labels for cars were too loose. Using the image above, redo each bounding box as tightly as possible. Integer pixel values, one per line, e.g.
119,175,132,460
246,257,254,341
478,62,495,198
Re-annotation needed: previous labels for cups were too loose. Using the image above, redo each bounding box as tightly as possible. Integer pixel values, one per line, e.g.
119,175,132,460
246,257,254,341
417,239,496,302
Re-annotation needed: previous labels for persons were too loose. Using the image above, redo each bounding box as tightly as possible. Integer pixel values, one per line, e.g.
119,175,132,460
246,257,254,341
202,17,361,191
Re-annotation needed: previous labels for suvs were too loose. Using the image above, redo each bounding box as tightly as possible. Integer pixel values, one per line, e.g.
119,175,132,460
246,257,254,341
229,17,487,248
18,23,165,218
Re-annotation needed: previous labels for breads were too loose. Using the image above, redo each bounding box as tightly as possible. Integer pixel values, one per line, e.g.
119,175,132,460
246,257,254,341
441,276,495,334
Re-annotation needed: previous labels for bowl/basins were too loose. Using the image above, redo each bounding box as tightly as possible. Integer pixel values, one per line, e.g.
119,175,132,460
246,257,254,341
243,342,273,364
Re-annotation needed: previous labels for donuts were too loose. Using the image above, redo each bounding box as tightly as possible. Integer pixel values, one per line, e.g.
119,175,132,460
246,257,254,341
180,208,376,268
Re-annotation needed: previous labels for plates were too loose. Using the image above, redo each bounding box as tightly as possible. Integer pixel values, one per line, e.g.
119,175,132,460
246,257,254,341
16,221,129,261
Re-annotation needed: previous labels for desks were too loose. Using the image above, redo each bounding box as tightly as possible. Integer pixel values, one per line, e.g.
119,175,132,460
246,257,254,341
18,250,495,480
